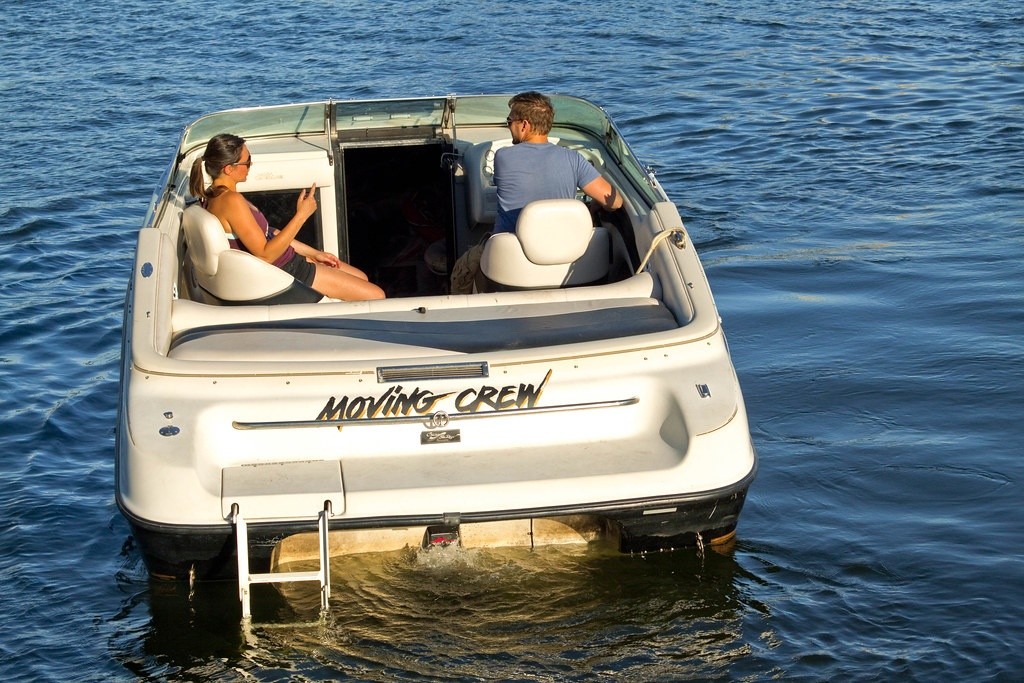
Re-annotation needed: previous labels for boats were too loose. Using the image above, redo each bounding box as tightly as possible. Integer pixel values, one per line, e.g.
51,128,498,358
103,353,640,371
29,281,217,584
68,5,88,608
113,93,760,632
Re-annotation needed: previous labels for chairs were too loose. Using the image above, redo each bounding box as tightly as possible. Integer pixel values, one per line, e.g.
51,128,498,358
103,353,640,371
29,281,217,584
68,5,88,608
472,199,613,295
183,205,341,307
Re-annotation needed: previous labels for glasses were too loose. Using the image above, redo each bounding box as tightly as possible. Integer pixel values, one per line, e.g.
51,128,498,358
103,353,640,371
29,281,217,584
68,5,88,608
507,117,521,128
233,156,251,168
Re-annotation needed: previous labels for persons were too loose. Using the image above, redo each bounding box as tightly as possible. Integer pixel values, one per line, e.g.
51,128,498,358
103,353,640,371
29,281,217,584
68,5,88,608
189,134,386,302
451,92,623,295
